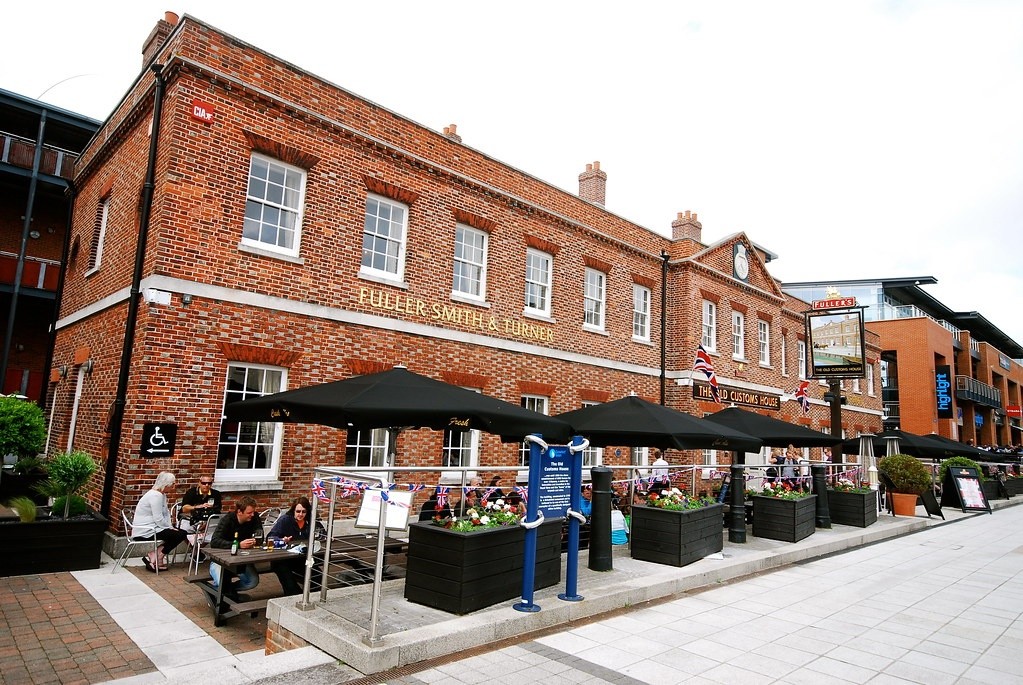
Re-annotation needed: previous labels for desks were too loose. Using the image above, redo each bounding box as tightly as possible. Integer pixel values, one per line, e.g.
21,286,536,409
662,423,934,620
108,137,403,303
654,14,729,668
200,533,408,627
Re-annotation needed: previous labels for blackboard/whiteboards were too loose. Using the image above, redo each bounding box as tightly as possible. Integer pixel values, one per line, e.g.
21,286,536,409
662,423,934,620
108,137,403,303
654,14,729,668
354,487,415,531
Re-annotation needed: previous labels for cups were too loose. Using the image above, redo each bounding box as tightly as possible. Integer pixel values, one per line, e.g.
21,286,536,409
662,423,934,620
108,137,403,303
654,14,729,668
208,498,214,507
267,537,274,553
252,529,263,547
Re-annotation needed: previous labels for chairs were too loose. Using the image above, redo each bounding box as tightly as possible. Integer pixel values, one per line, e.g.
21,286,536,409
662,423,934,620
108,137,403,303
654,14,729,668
171,501,282,576
111,508,169,576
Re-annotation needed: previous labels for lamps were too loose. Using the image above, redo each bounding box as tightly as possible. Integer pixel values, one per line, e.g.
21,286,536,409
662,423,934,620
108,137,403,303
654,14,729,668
58,364,67,376
81,360,93,374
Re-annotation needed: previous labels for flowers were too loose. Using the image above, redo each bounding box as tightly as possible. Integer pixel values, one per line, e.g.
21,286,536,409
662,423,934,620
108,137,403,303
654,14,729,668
432,499,527,533
744,482,809,500
646,483,716,511
1007,470,1023,478
832,479,871,496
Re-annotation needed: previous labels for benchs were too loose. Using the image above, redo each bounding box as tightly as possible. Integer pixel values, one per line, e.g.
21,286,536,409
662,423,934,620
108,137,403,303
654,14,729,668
231,598,269,614
182,554,361,583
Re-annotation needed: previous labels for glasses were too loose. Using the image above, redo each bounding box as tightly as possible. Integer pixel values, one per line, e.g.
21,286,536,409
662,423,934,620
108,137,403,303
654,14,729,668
825,452,827,454
296,510,307,513
200,481,212,485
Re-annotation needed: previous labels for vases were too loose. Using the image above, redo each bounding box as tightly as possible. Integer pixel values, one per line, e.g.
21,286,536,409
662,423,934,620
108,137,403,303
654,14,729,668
404,515,565,616
827,488,878,528
752,494,818,543
1007,478,1023,494
630,503,725,567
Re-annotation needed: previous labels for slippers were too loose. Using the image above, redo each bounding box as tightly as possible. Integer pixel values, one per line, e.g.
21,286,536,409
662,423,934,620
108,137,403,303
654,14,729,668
142,556,168,573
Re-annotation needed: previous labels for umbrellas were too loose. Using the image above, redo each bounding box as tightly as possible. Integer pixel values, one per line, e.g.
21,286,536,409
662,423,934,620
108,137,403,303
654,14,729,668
551,390,763,468
843,426,980,459
922,430,1007,463
702,402,844,465
225,362,578,536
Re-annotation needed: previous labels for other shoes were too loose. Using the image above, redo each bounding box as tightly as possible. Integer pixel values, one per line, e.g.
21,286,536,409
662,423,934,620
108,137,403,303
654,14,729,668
189,553,207,563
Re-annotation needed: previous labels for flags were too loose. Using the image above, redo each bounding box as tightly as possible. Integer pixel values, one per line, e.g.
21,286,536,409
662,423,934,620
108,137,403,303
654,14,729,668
480,488,498,502
692,344,721,404
795,381,810,414
612,472,682,491
463,487,477,501
310,476,451,508
513,485,528,503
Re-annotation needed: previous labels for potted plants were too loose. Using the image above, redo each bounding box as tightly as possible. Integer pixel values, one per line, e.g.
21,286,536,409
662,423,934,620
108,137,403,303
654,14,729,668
878,453,932,516
983,477,999,500
0,397,108,578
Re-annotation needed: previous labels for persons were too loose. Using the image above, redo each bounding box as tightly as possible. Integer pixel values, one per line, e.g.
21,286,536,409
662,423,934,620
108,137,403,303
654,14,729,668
582,481,592,524
267,497,328,595
131,470,264,600
769,443,833,490
418,485,452,521
647,452,670,494
965,438,1023,455
454,476,527,518
632,493,647,505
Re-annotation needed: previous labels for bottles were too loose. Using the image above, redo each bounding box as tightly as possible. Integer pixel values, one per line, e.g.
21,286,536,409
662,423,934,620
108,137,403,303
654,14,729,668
230,532,239,556
